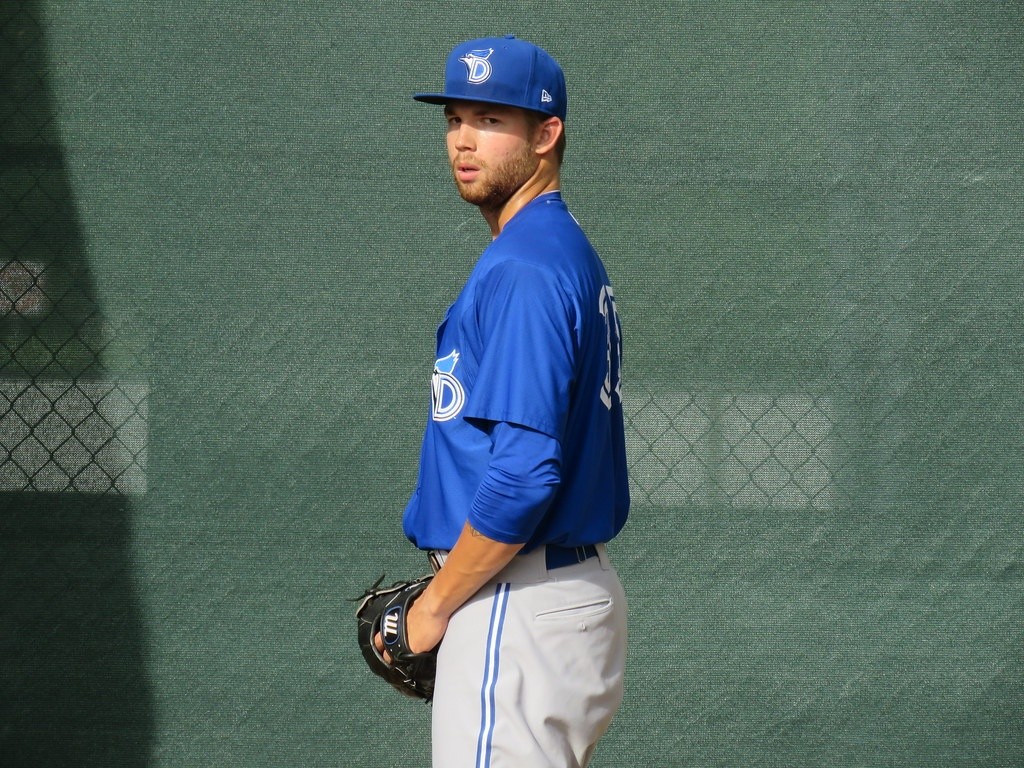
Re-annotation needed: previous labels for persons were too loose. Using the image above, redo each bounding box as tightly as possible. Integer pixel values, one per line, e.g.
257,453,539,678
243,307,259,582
355,34,631,768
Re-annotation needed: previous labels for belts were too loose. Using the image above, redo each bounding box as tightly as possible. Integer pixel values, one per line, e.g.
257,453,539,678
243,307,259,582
427,543,598,570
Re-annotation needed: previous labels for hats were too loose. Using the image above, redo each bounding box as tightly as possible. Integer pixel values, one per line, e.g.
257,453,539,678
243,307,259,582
412,34,567,120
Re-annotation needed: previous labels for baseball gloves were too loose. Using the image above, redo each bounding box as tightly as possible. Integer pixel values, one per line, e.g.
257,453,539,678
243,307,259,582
341,572,439,706
